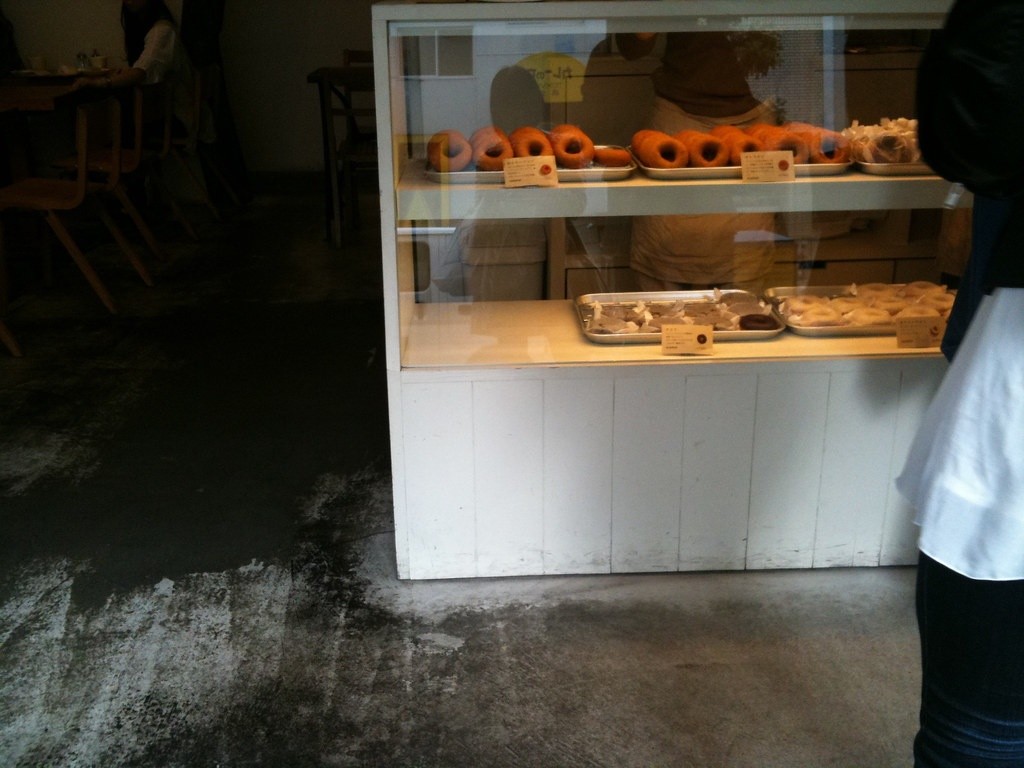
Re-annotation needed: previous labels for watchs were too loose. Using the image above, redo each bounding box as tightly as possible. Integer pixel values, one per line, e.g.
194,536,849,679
105,77,112,88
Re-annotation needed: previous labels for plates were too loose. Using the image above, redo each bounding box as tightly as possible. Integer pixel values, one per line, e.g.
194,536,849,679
81,68,109,77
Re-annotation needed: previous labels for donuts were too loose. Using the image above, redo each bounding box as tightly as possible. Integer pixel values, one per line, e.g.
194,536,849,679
427,122,632,172
632,121,853,170
843,123,925,164
783,280,957,327
587,291,778,334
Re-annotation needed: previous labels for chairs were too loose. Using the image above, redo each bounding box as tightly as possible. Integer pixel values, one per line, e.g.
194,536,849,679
138,68,219,244
76,90,202,268
321,48,374,246
0,99,156,315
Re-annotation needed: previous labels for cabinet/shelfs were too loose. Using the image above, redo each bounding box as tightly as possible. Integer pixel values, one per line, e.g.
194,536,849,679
544,58,971,285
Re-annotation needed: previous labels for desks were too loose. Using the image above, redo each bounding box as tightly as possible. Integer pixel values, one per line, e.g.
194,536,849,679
1,77,119,292
306,64,377,239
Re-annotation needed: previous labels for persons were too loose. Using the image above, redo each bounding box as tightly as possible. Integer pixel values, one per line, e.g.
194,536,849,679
617,31,779,291
912,0,1024,768
74,0,215,177
